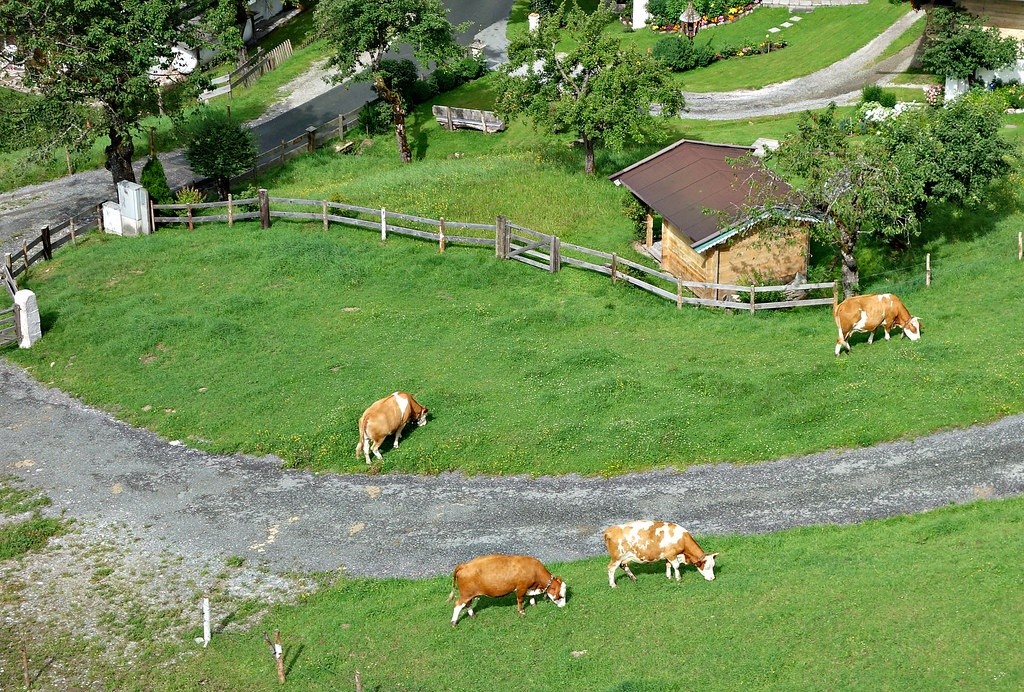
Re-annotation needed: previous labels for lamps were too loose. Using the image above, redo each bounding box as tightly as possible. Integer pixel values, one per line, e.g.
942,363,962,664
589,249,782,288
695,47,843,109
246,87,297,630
678,2,702,39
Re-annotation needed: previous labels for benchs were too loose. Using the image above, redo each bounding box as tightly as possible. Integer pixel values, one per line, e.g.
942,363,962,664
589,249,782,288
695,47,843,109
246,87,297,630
432,104,506,135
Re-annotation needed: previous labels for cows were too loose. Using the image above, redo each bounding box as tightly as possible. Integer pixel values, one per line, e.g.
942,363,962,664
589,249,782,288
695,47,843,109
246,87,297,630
445,555,567,628
603,520,719,588
834,292,923,357
356,391,430,464
